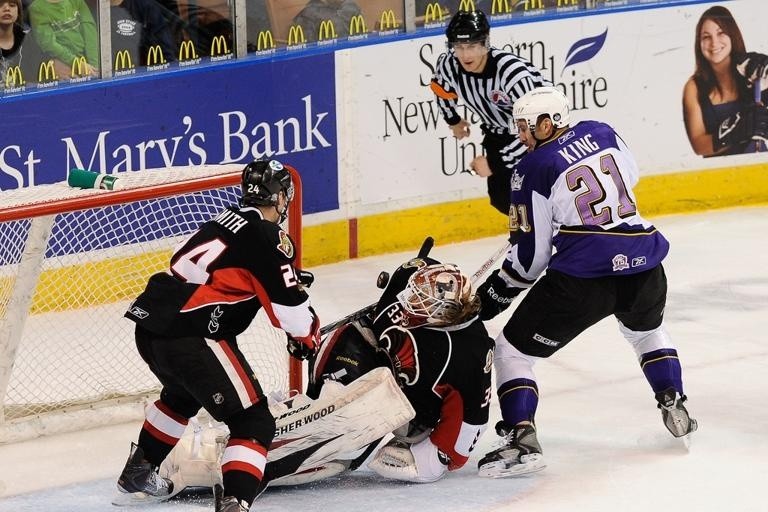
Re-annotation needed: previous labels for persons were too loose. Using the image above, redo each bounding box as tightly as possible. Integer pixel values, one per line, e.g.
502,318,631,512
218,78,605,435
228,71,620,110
288,1,365,44
0,0,73,89
682,6,767,160
430,9,545,215
28,0,100,80
475,86,698,467
115,158,320,512
142,4,259,65
305,263,495,484
110,0,143,71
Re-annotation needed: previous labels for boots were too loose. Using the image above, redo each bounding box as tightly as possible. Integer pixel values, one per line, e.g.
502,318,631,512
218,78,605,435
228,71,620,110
117,442,173,497
213,482,249,512
655,386,696,436
477,412,542,471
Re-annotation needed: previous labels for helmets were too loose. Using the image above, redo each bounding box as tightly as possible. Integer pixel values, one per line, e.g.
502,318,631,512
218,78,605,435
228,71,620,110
445,10,489,56
240,160,294,208
513,87,571,130
401,265,471,329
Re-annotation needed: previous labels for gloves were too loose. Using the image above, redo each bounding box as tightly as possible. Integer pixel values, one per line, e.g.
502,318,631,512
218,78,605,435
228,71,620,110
737,52,768,90
711,100,767,151
475,270,527,321
287,306,321,360
